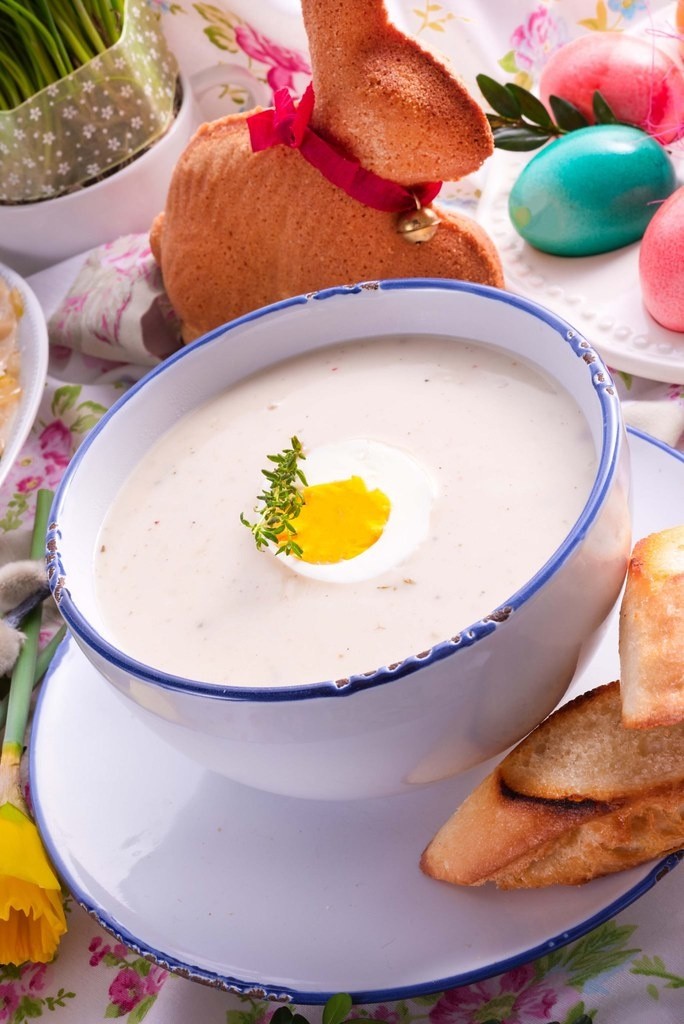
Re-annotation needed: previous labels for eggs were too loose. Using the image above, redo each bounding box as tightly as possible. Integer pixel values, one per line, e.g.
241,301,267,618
255,444,432,584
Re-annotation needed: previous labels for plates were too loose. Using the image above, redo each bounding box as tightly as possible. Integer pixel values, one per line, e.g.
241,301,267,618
0,260,48,481
473,0,684,383
28,424,684,1003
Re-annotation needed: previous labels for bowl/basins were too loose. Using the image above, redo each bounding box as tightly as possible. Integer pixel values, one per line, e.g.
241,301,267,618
46,277,632,801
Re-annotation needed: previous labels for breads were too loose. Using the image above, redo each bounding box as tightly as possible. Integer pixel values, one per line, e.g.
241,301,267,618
146,1,507,348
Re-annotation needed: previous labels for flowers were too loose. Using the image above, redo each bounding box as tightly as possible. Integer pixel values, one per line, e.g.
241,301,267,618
0,487,68,968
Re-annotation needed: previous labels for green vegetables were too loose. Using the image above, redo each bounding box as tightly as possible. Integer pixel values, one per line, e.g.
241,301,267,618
239,439,308,559
